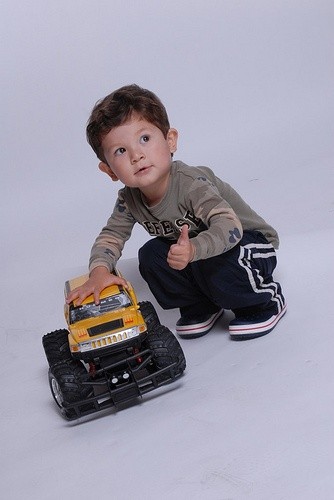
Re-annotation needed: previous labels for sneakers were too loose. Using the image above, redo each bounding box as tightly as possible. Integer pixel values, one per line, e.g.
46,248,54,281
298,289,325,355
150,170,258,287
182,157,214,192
176,308,224,337
228,304,287,337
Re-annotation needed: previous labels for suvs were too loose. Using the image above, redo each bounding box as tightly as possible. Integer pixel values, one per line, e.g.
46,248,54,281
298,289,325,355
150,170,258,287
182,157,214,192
41,266,189,423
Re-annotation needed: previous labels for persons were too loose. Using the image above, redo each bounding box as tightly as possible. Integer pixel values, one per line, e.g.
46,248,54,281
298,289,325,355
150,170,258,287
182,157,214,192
65,83,288,341
